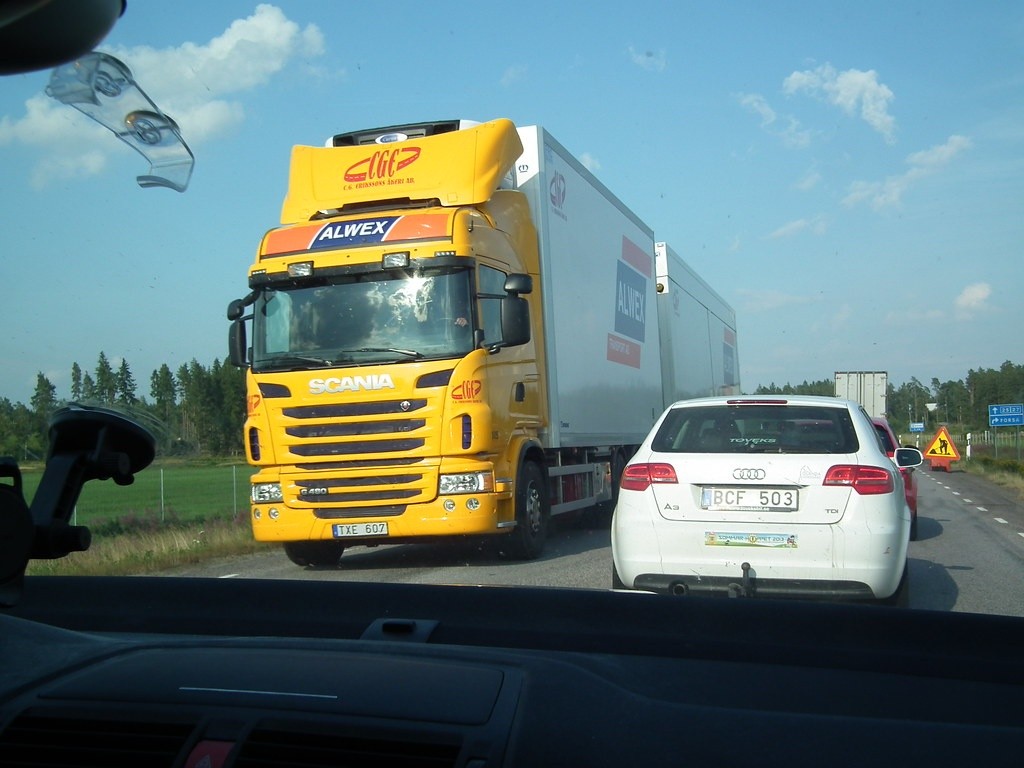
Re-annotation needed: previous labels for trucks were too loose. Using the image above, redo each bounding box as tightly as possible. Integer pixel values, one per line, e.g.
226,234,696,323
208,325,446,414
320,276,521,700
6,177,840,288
834,369,887,416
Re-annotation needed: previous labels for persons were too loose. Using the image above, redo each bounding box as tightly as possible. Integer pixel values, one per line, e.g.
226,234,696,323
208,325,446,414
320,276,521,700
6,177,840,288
453,314,469,329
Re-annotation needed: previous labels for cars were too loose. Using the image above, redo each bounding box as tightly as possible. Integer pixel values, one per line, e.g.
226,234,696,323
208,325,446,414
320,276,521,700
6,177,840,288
610,394,923,608
870,418,918,541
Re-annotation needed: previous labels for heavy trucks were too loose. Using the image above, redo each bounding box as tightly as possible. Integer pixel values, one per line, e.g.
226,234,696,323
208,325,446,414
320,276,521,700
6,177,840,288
228,119,741,566
